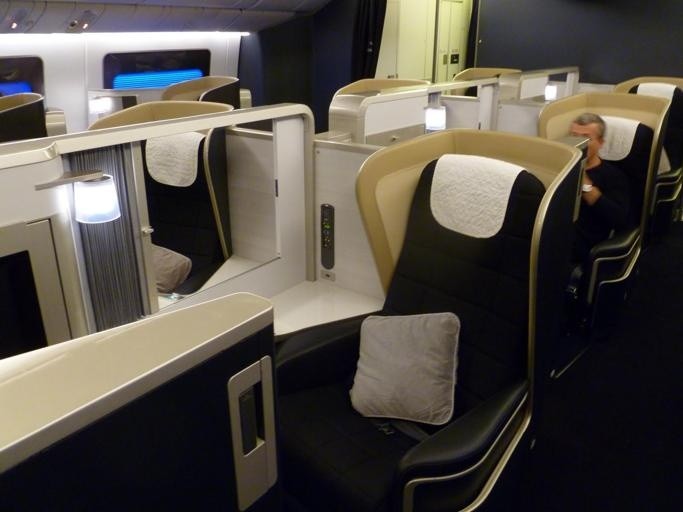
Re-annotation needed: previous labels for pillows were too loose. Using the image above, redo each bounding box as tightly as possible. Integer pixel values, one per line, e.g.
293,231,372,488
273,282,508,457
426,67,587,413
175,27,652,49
350,312,460,425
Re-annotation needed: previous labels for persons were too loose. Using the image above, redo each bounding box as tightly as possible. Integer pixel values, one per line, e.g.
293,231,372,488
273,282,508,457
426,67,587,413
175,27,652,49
549,112,628,336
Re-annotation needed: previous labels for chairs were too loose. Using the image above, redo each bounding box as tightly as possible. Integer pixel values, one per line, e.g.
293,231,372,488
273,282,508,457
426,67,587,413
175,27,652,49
616,76,683,219
271,128,583,512
3,67,521,358
537,90,673,323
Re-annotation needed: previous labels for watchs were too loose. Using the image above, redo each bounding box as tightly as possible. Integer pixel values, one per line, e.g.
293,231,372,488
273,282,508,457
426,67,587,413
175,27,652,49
582,182,596,192
